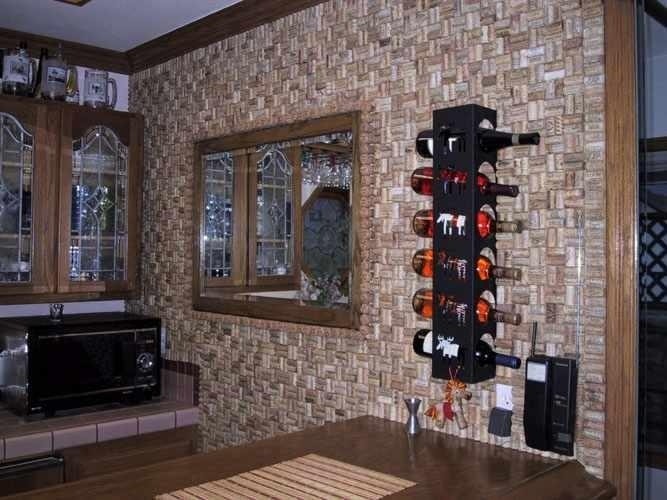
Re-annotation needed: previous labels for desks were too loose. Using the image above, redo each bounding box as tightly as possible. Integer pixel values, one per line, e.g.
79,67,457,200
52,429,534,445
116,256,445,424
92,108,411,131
0,414,620,496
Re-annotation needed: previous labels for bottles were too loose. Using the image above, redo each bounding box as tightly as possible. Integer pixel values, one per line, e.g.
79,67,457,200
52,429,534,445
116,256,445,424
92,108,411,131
413,329,521,370
412,209,521,241
414,127,542,159
408,166,519,201
412,248,522,287
411,288,521,329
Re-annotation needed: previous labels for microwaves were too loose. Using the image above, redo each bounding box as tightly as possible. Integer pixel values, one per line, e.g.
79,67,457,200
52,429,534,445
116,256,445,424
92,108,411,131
0,311,162,421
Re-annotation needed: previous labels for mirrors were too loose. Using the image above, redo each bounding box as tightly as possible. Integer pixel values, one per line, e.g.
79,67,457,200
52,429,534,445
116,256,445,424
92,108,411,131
195,109,360,332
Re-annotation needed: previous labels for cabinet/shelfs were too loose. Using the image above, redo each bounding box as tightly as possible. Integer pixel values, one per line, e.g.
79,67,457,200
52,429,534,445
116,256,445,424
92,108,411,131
202,143,303,295
0,95,144,301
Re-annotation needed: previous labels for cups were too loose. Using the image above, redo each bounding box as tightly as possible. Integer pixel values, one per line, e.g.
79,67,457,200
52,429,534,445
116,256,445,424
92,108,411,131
40,57,78,101
2,55,38,96
83,69,118,110
